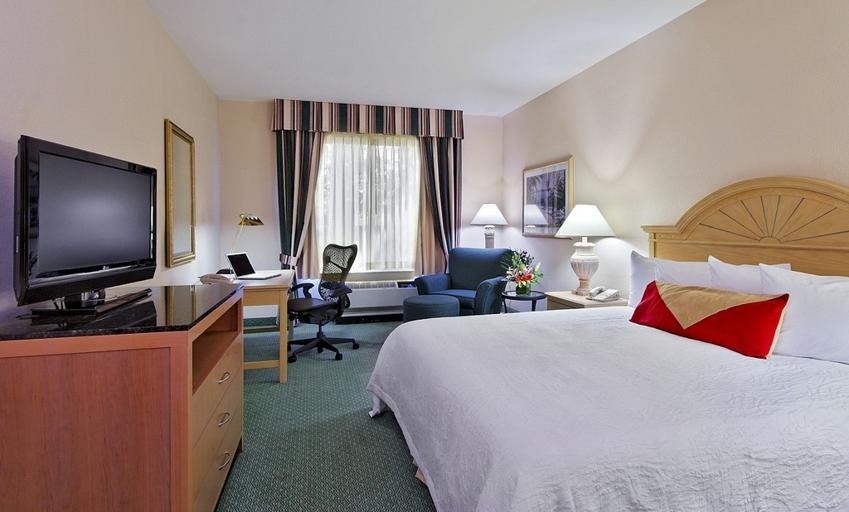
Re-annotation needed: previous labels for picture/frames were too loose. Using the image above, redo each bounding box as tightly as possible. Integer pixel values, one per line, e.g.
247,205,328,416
522,154,575,240
164,118,196,268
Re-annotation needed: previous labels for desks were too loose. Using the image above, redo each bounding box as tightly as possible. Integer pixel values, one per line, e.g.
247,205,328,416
190,269,297,385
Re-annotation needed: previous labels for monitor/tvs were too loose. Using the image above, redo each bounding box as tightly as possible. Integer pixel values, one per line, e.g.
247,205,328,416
14,135,157,314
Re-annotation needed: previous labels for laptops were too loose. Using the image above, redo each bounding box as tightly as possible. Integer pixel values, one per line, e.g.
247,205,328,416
226,252,281,280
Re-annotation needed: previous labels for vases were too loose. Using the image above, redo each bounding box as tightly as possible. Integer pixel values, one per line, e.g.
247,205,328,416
515,285,531,295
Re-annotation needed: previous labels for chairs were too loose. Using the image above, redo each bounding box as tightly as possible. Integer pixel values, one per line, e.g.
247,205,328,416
276,244,360,363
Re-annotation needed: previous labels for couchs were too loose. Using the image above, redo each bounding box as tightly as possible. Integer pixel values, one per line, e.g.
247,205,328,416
415,247,513,316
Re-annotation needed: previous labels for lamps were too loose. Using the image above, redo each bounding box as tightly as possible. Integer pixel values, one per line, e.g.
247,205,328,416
215,212,265,274
554,204,616,295
470,203,508,248
523,203,549,228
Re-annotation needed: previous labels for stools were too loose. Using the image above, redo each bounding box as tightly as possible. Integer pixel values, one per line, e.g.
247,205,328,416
403,294,460,323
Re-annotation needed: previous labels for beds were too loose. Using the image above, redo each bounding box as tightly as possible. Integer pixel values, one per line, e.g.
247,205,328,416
366,177,847,511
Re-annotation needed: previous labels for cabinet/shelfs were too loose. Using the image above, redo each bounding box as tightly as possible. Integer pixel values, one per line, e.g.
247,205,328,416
0,283,245,512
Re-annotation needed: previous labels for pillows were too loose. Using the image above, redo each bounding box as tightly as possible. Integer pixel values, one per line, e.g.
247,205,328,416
628,279,790,360
758,261,847,365
708,255,791,297
628,250,708,310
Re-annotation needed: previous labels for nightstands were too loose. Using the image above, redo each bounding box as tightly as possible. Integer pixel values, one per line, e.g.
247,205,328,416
544,291,628,311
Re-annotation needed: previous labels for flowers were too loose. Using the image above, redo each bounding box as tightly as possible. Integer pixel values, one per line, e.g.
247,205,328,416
500,247,544,287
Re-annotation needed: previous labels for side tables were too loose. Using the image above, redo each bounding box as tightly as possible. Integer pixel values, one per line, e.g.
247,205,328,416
500,291,547,313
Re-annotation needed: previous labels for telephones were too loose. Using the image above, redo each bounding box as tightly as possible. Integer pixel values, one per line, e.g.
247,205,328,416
200,274,232,285
586,286,620,301
